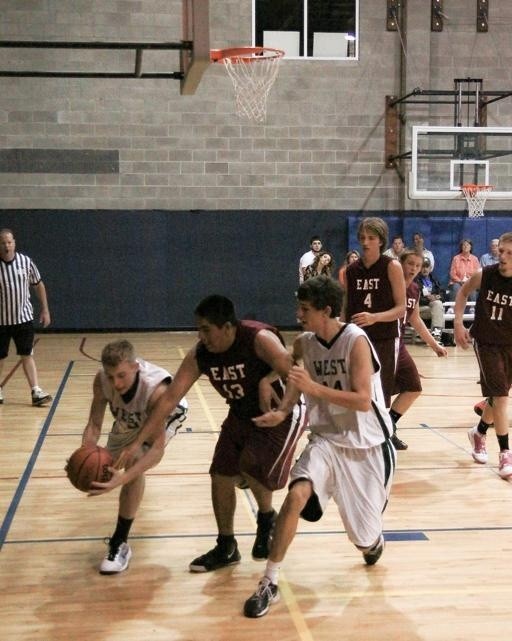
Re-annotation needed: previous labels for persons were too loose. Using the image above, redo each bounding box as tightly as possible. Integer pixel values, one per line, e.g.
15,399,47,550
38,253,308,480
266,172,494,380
0,228,53,406
298,231,501,346
244,274,397,619
474,397,512,428
64,339,189,576
388,251,449,450
454,232,512,481
113,294,302,572
341,216,407,408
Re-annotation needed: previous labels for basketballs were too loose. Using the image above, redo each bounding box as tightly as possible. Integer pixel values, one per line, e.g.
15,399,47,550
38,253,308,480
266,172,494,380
67,446,113,492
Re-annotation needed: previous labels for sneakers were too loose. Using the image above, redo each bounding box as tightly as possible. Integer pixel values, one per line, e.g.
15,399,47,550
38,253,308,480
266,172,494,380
189,510,282,618
499,449,512,478
32,388,52,406
468,425,487,464
390,423,407,450
100,538,132,573
356,535,384,565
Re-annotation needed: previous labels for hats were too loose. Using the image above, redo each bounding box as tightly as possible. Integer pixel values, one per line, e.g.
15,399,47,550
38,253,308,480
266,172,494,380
422,257,430,266
